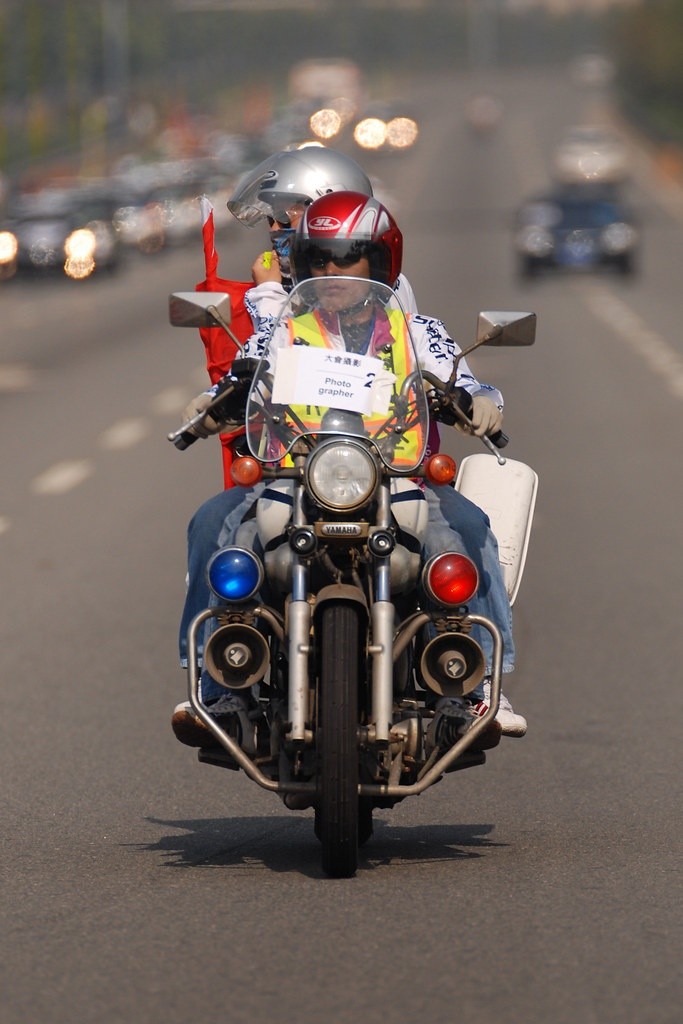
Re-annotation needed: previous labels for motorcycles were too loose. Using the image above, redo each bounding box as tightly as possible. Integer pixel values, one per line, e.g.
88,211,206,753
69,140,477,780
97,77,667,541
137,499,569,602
164,286,538,878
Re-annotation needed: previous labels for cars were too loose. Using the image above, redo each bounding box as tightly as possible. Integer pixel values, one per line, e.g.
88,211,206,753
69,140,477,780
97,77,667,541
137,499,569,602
0,136,272,284
516,193,635,284
554,123,629,191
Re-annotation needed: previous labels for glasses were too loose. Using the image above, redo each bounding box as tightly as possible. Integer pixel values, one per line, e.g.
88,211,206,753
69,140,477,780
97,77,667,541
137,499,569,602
266,206,303,229
305,247,371,270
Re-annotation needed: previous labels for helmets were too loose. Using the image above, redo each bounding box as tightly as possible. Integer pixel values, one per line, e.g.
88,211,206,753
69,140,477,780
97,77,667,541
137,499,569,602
227,145,373,249
288,190,402,302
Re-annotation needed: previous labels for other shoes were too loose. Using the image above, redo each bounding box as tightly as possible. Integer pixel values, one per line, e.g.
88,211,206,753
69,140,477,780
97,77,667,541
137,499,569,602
440,702,503,752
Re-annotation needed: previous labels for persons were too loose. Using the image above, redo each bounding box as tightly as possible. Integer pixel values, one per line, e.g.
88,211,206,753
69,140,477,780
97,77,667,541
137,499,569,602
171,146,526,772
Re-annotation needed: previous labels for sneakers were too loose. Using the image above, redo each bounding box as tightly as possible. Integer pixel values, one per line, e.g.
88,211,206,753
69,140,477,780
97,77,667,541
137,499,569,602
171,699,240,758
463,676,527,740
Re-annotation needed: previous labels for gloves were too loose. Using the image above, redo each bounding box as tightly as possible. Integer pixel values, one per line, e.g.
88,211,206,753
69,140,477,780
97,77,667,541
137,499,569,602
455,395,503,437
181,395,222,440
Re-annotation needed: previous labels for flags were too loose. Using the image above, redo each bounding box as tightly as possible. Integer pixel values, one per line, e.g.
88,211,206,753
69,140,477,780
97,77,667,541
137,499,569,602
195,204,260,490
